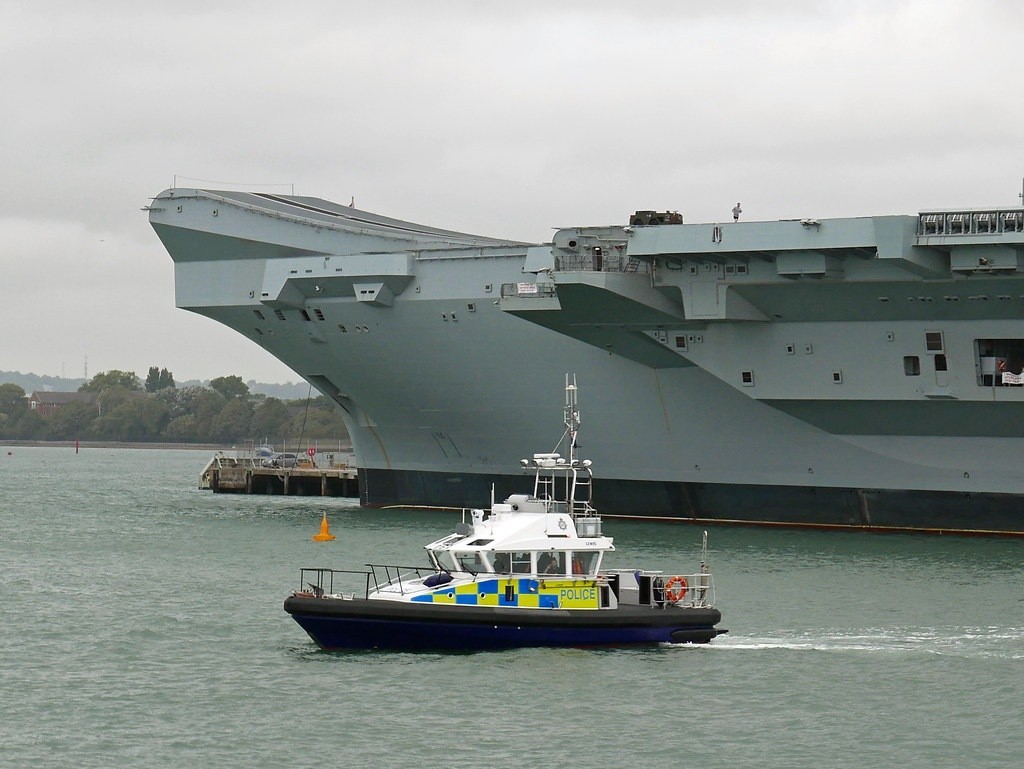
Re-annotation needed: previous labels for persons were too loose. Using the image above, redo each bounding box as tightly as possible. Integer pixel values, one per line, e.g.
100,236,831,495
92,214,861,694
490,551,566,579
732,203,742,222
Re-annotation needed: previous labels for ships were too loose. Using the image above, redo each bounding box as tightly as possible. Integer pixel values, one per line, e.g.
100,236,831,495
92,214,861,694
140,175,1024,536
282,372,730,655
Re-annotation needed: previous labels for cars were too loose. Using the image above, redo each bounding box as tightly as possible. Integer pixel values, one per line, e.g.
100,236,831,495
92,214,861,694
262,453,300,469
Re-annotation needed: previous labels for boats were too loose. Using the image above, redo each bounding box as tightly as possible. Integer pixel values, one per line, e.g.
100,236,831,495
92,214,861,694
254,438,275,456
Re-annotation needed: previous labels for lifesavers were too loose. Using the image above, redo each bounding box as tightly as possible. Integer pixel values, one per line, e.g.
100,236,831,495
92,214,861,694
666,577,688,601
